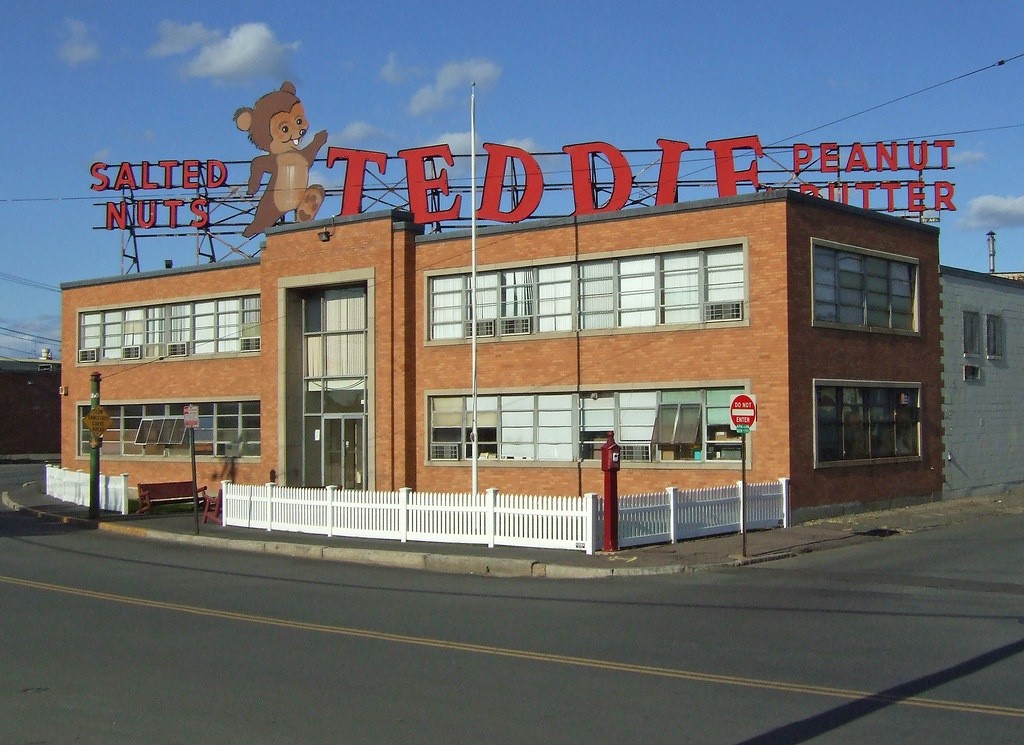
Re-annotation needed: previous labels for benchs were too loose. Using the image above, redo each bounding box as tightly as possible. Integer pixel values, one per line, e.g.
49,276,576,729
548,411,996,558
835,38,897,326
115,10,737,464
136,480,207,516
203,488,222,525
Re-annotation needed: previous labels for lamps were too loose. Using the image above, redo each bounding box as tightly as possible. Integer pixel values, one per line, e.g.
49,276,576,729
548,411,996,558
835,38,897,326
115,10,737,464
166,259,173,269
318,231,332,242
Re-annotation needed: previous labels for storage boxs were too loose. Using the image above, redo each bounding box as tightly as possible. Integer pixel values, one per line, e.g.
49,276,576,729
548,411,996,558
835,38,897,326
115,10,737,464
714,431,728,441
663,450,675,461
594,437,611,459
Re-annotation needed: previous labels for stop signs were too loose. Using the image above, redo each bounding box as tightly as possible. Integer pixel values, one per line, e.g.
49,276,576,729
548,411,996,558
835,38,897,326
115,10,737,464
729,394,757,431
